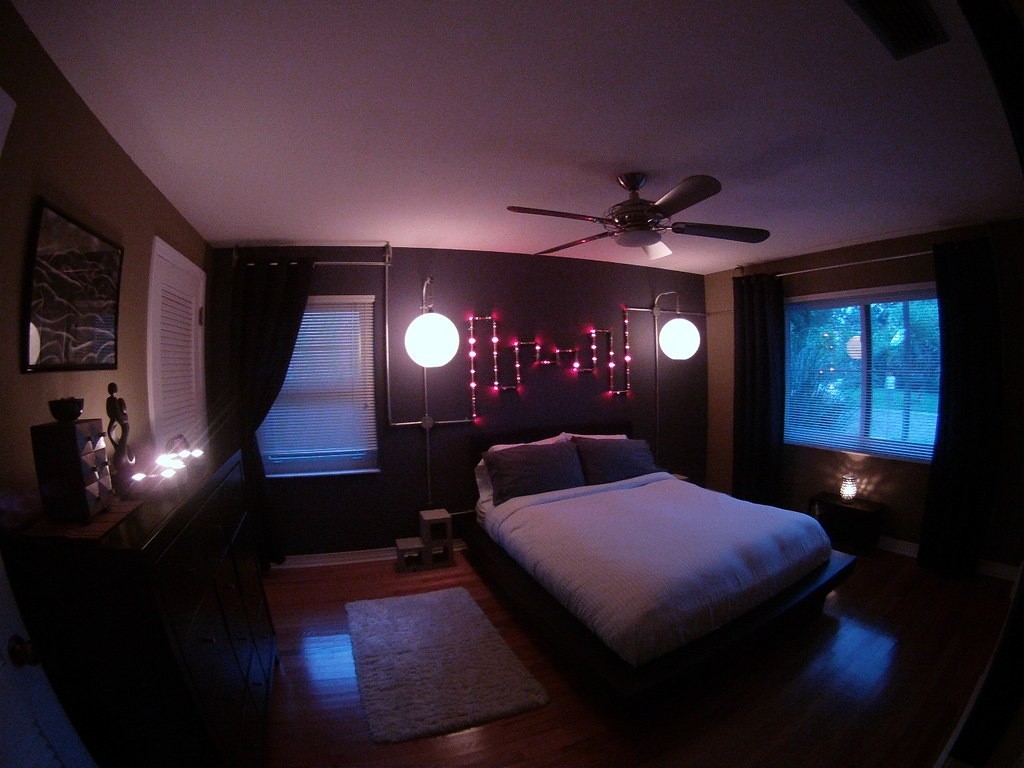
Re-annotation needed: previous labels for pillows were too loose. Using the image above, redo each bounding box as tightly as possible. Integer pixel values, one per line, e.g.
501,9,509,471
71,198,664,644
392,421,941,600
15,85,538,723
474,431,567,505
570,435,657,485
565,433,628,441
481,440,586,507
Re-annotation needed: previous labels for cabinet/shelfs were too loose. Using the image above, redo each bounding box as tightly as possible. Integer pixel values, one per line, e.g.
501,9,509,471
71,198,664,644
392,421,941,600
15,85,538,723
0,448,282,768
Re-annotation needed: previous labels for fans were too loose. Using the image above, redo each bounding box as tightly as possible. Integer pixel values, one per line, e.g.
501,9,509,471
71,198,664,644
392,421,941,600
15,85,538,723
506,172,771,257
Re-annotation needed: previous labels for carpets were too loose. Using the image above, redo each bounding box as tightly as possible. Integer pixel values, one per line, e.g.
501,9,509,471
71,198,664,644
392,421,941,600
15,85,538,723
344,588,550,744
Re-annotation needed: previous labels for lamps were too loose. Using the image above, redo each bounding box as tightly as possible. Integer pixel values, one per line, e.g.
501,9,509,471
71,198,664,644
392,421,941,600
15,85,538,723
132,449,205,493
840,471,858,505
652,290,701,468
404,276,461,507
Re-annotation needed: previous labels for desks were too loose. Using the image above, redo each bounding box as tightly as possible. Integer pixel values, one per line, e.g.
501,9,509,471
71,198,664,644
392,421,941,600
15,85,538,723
808,489,882,558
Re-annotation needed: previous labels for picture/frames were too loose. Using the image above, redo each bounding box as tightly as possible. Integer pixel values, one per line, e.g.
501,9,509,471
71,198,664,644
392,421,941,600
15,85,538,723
19,194,125,375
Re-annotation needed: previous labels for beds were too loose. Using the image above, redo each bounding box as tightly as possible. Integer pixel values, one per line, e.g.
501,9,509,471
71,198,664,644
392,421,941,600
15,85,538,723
462,420,858,738
